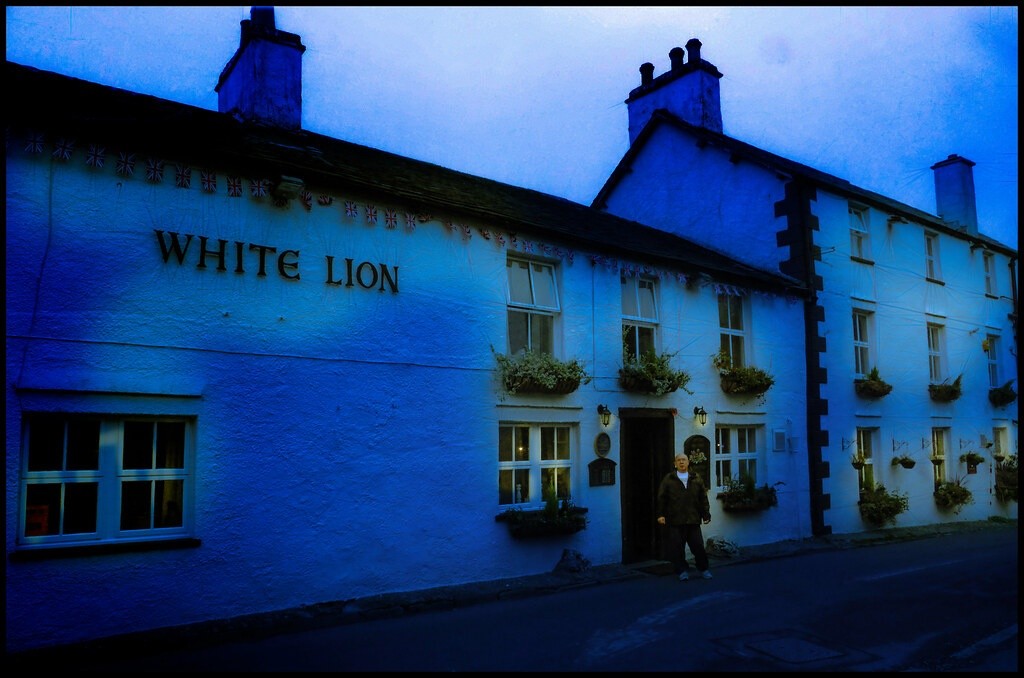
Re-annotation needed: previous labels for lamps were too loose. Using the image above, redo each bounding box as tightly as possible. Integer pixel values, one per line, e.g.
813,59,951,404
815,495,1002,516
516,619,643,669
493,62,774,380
599,403,612,425
694,406,707,425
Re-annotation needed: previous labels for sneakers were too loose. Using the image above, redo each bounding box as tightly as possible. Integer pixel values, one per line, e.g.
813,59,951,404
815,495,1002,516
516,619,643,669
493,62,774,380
679,572,689,583
702,570,712,579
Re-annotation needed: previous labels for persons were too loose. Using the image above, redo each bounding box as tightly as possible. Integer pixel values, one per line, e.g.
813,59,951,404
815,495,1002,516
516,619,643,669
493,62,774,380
657,453,714,580
